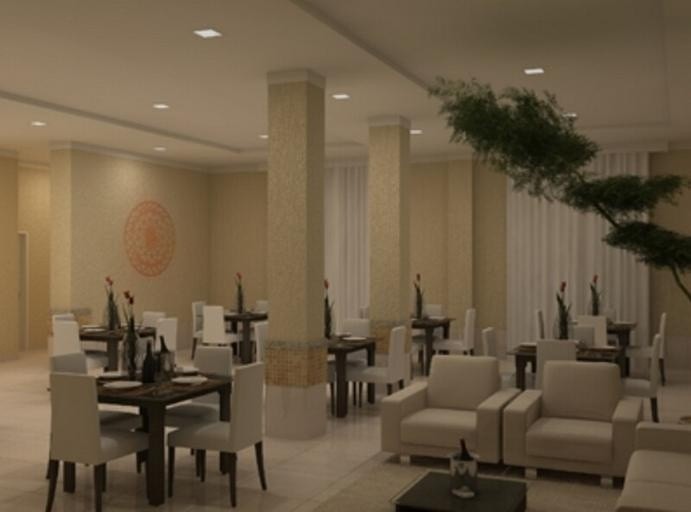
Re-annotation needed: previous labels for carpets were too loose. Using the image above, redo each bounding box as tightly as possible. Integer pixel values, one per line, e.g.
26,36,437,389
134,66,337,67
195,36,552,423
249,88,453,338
281,449,624,511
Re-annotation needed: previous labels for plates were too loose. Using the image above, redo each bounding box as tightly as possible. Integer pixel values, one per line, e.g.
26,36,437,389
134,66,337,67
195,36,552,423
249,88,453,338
174,366,200,373
169,376,208,384
335,332,352,336
590,345,616,351
343,337,366,340
82,325,100,328
521,342,538,347
103,380,143,390
98,371,128,378
85,329,106,333
429,316,447,321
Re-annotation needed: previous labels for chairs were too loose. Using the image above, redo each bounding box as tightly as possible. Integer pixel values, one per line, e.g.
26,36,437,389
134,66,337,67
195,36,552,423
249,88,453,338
578,316,607,345
482,327,518,385
536,310,544,339
535,339,576,391
625,312,667,385
621,333,661,422
327,303,477,417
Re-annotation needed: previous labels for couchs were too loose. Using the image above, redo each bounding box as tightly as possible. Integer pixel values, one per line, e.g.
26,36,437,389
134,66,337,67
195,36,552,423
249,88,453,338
381,355,521,465
614,422,691,512
504,360,643,489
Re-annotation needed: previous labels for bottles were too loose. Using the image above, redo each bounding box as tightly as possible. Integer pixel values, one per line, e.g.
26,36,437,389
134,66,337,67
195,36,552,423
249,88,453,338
126,336,176,383
456,439,472,461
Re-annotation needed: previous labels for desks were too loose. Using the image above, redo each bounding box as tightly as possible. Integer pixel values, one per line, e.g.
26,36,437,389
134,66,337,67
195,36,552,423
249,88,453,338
607,323,637,344
506,344,619,389
395,471,526,512
